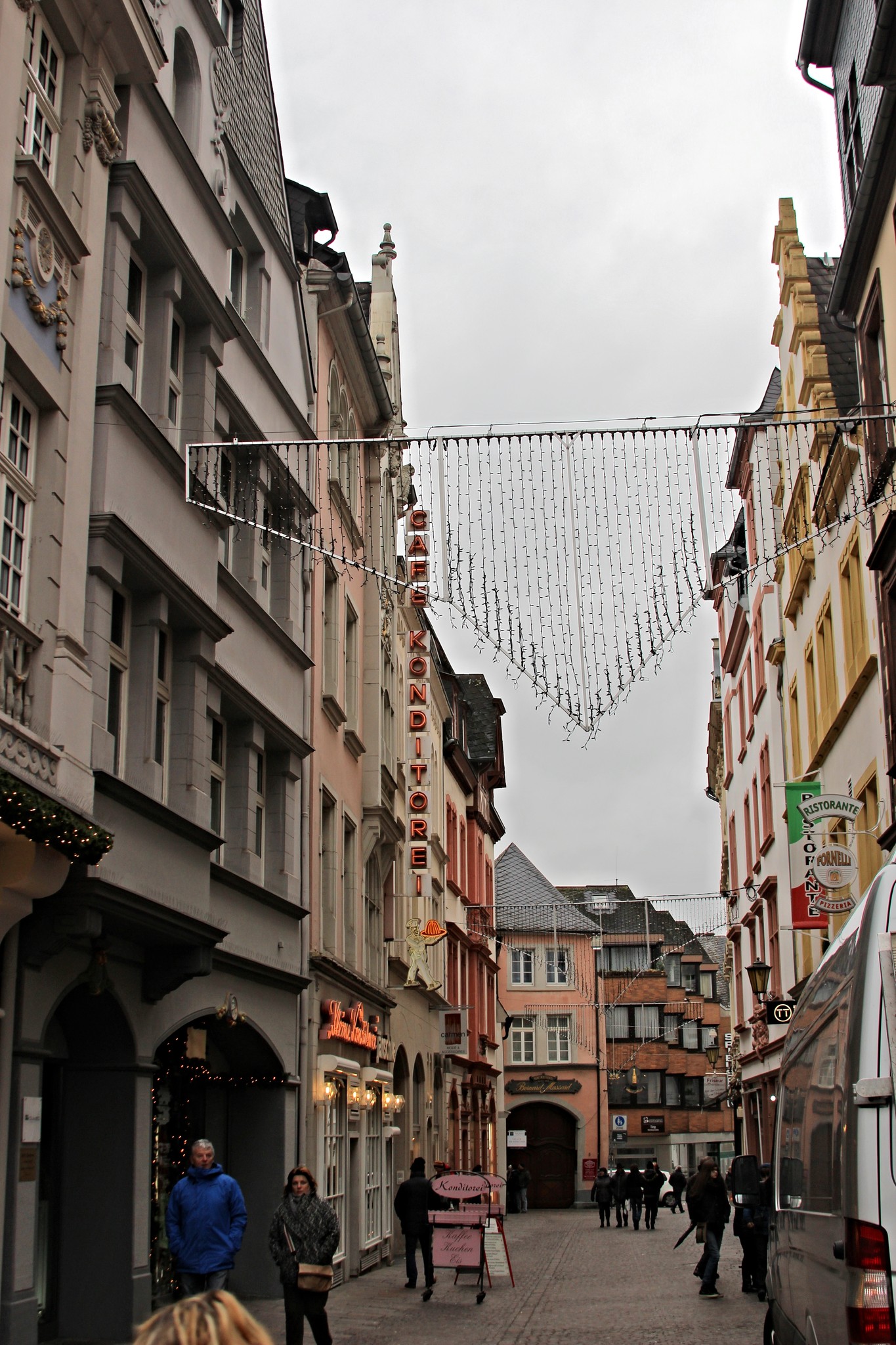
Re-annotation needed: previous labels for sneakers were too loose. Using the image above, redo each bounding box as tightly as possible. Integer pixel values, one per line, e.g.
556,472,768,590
426,1277,436,1287
693,1265,767,1302
405,1278,416,1288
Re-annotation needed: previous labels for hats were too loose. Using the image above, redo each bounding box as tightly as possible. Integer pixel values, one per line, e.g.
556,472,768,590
647,1161,654,1169
615,1163,624,1168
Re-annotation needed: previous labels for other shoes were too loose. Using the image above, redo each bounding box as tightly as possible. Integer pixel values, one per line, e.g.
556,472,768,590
521,1211,526,1213
598,1208,685,1230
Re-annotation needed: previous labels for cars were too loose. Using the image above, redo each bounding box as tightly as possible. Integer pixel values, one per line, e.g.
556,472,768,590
609,1170,678,1208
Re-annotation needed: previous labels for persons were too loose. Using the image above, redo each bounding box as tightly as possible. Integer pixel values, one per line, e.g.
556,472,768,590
271,1166,342,1345
591,1162,668,1231
669,1166,687,1214
132,1288,275,1345
687,1155,775,1302
393,1156,452,1290
164,1138,249,1300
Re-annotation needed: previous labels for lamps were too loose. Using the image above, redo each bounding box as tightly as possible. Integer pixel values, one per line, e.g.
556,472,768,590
705,1041,732,1072
745,957,772,1004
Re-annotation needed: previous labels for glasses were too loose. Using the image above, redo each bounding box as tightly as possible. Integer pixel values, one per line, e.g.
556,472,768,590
712,1169,718,1172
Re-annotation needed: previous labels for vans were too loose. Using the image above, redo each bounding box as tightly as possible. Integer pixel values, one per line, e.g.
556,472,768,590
758,857,896,1343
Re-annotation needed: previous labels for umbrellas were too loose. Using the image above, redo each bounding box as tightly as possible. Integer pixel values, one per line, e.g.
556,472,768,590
673,1223,696,1250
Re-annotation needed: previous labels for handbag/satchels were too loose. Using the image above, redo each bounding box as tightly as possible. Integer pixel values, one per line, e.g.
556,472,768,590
696,1222,707,1243
297,1263,333,1292
